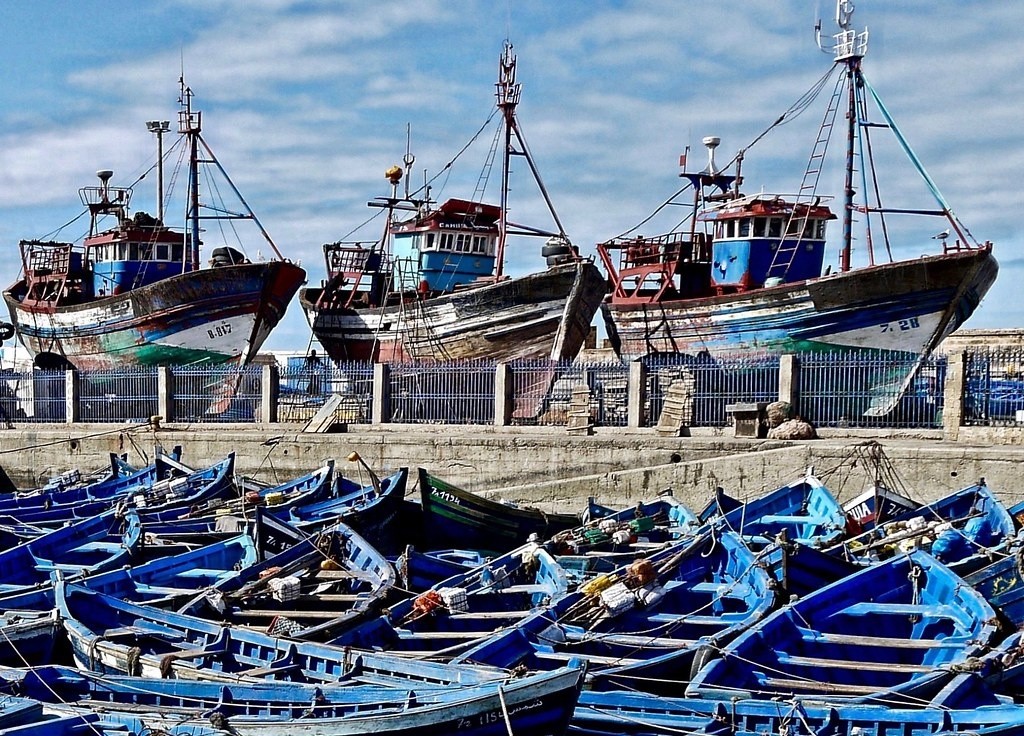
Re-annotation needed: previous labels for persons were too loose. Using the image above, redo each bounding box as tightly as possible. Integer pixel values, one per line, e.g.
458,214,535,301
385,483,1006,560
324,271,349,303
306,350,325,393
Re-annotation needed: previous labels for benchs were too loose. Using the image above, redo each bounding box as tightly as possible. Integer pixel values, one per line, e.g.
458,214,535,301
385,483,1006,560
665,580,752,601
588,515,827,556
646,610,742,627
533,644,645,666
374,647,437,657
450,610,531,620
330,506,354,514
756,673,890,693
237,664,299,677
398,628,492,640
490,583,548,594
0,505,238,736
566,627,699,649
324,678,358,688
301,567,370,581
231,605,347,618
775,651,934,673
846,603,971,630
299,592,371,601
804,628,965,650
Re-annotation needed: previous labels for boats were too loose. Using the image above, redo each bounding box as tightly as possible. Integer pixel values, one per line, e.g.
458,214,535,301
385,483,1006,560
2,55,308,421
0,444,1023,736
595,2,999,428
295,31,608,426
